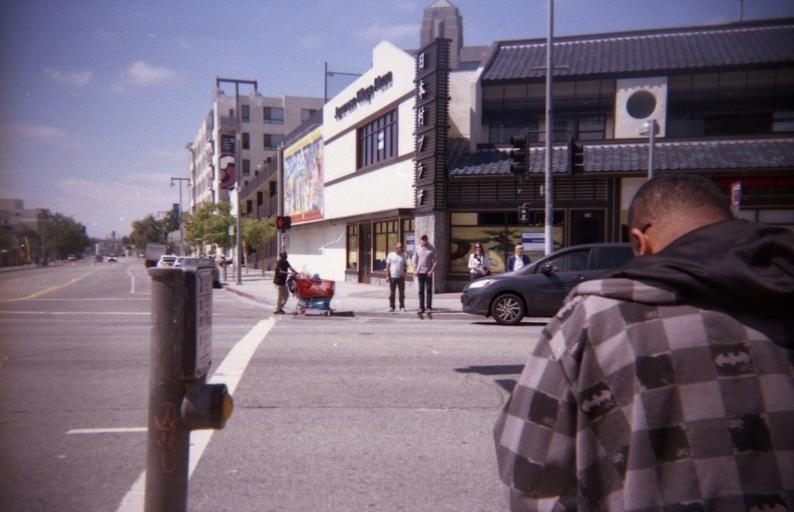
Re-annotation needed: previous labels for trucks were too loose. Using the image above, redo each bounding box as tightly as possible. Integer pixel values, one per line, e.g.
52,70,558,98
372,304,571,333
145,243,168,268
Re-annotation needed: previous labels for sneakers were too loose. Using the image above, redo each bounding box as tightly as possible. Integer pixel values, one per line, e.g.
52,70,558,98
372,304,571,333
388,308,432,314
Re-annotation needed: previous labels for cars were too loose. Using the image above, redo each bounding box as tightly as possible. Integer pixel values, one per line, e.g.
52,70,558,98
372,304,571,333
461,242,636,325
68,254,117,262
156,255,219,288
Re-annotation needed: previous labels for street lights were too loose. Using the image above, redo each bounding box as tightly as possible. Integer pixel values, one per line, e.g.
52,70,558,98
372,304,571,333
209,76,263,285
639,119,659,182
171,178,192,256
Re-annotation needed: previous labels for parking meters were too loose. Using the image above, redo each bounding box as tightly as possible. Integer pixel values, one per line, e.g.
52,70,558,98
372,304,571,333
142,254,234,512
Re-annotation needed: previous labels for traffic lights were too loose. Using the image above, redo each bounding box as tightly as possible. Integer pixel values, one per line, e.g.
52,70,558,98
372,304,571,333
275,216,291,230
566,139,585,177
509,130,529,185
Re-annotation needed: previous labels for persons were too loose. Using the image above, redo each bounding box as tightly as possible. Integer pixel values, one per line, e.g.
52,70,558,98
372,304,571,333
506,245,531,272
385,241,407,312
411,235,438,315
467,241,490,280
272,250,299,314
493,168,794,512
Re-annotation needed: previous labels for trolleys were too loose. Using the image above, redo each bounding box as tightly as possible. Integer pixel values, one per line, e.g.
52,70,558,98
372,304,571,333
288,272,335,316
